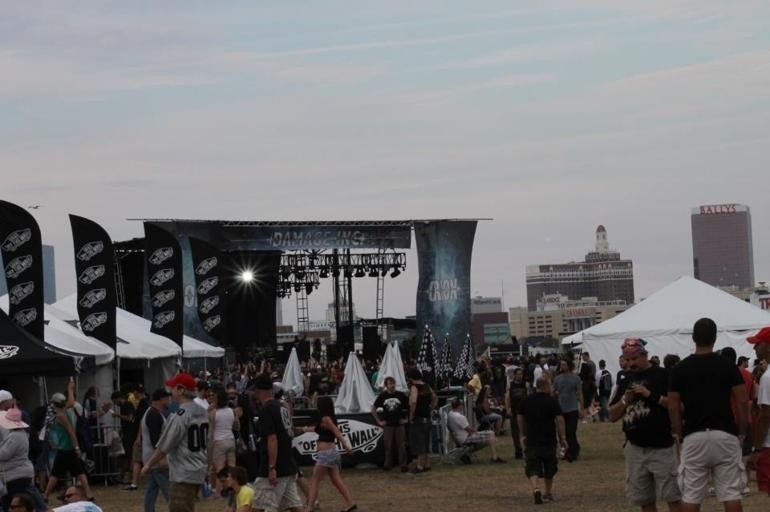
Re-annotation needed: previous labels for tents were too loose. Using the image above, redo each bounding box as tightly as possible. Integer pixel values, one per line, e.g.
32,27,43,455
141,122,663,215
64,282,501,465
562,274,770,396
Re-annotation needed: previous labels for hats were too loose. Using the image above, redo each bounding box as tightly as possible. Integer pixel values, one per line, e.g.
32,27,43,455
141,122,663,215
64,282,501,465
0,390,29,430
747,327,770,343
49,393,66,403
256,373,272,389
152,373,195,401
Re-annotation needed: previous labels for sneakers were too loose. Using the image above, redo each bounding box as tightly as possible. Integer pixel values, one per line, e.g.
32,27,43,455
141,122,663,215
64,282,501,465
534,489,542,504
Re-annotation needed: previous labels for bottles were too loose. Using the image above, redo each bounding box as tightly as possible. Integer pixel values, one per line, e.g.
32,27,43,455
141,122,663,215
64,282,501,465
559,444,566,457
201,480,212,499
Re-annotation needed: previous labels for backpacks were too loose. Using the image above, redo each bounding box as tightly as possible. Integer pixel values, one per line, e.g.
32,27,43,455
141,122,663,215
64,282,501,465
74,408,91,449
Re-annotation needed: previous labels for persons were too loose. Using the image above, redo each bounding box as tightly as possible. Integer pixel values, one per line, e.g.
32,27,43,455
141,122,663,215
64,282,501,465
0,324,769,512
668,316,749,512
